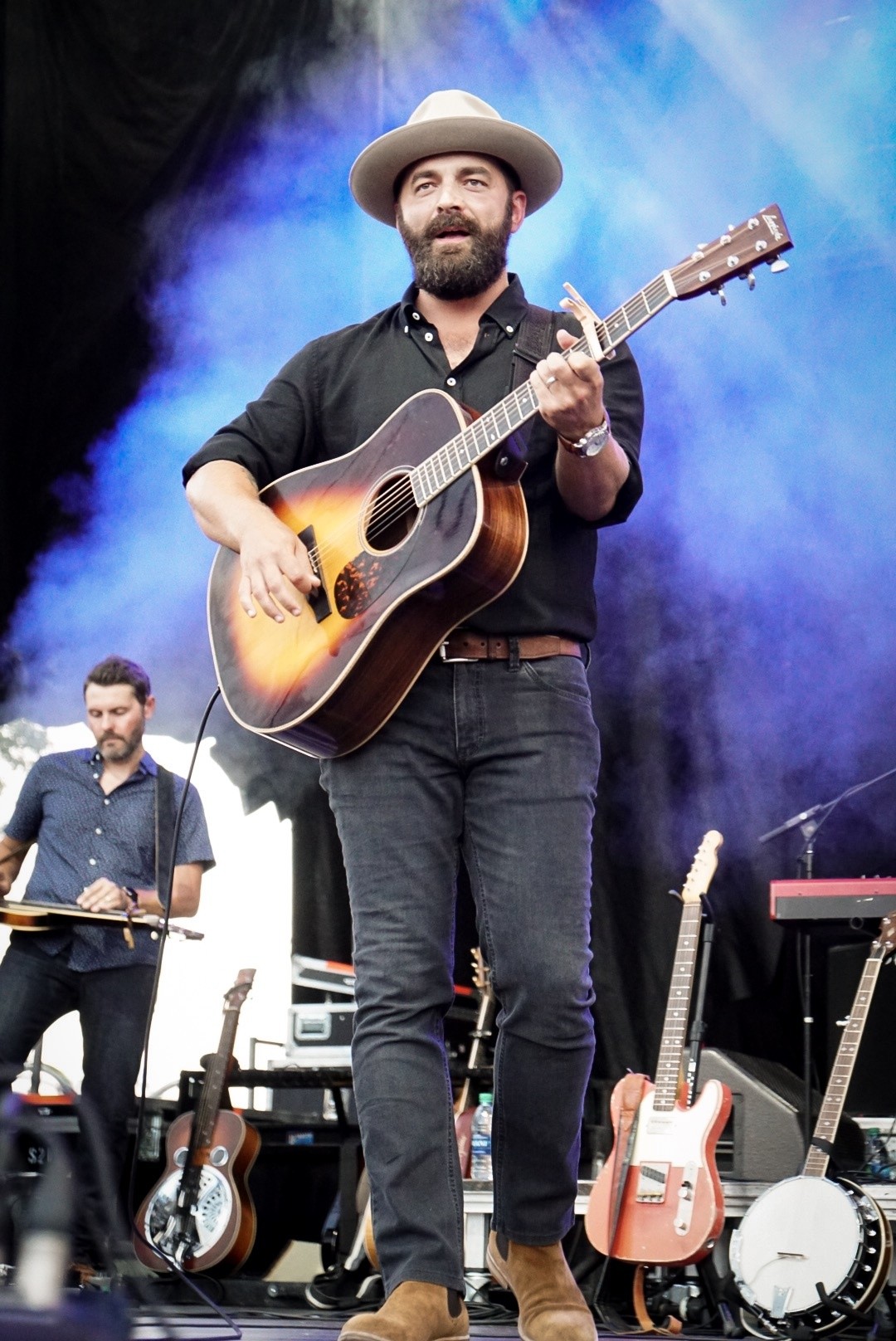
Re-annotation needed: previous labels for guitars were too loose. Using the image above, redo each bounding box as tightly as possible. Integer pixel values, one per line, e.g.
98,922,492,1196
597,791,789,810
583,829,736,1265
365,945,498,1263
724,911,896,1340
0,898,205,942
132,968,262,1281
206,201,794,761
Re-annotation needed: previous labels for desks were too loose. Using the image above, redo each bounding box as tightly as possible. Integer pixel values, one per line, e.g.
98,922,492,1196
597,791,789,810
176,1066,618,1160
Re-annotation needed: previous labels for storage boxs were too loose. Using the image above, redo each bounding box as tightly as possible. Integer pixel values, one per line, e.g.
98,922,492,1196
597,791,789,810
289,964,481,1068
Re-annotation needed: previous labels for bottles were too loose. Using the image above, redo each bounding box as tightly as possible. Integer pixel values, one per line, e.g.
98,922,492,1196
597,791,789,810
471,1091,492,1185
864,1128,890,1177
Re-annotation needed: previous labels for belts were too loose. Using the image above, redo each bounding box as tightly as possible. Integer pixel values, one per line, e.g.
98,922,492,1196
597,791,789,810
432,626,582,663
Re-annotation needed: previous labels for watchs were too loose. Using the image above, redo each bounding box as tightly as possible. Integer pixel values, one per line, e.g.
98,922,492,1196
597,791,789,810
558,408,614,460
122,886,139,909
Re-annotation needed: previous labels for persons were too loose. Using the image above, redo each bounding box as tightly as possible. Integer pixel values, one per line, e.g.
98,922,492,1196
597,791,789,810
178,86,648,1341
0,653,215,1212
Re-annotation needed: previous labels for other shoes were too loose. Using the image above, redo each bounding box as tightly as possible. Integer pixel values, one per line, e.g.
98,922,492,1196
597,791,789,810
305,1257,385,1311
338,1280,469,1341
65,1261,112,1292
486,1228,598,1341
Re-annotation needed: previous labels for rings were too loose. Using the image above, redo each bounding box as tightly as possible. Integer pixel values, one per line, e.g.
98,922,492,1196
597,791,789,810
545,375,557,388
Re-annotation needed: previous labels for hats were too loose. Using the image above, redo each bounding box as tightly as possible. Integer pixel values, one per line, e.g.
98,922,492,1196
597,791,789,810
349,89,563,228
